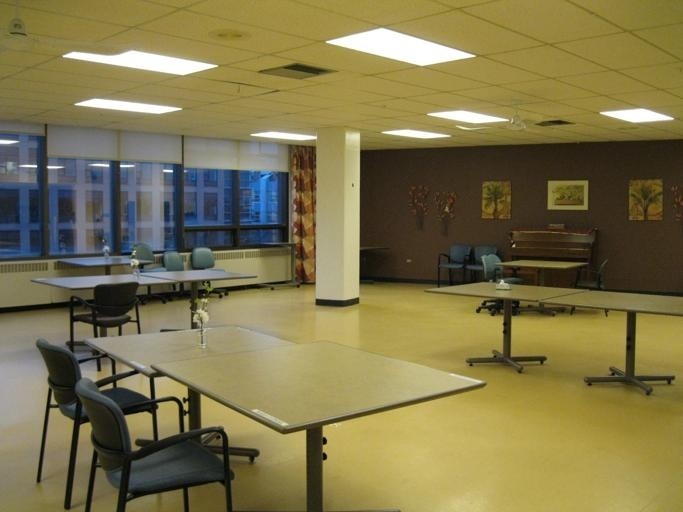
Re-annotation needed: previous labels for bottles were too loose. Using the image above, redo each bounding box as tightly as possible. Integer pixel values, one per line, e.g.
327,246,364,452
130,249,141,282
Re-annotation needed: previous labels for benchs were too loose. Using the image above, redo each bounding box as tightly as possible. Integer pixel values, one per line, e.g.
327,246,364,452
491,227,599,288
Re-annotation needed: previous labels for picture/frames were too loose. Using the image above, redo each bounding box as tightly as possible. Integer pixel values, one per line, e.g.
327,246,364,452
546,180,589,211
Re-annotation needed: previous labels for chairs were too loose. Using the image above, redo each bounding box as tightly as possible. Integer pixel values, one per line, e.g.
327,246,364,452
34,338,234,511
569,259,609,317
437,245,523,316
423,281,683,395
129,243,228,305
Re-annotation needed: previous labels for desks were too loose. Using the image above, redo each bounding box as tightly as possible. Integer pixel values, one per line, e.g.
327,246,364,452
29,256,257,347
494,259,589,316
